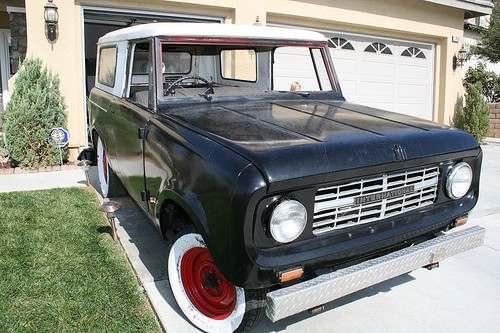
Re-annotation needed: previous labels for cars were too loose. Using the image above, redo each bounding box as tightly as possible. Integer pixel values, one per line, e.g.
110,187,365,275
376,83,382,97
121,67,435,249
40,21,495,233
86,22,486,333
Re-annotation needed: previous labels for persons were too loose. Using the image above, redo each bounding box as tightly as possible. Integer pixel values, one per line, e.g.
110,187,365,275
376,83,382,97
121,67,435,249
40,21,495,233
290,81,300,90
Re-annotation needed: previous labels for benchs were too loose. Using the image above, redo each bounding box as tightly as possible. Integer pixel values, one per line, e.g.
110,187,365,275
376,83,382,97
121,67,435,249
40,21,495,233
133,86,255,107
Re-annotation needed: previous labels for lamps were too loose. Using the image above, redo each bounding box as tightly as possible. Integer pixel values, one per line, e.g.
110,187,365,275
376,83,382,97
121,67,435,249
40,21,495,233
453,44,467,70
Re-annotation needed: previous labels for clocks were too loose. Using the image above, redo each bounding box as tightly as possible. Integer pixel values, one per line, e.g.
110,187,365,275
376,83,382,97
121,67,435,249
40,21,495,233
44,0,58,41
